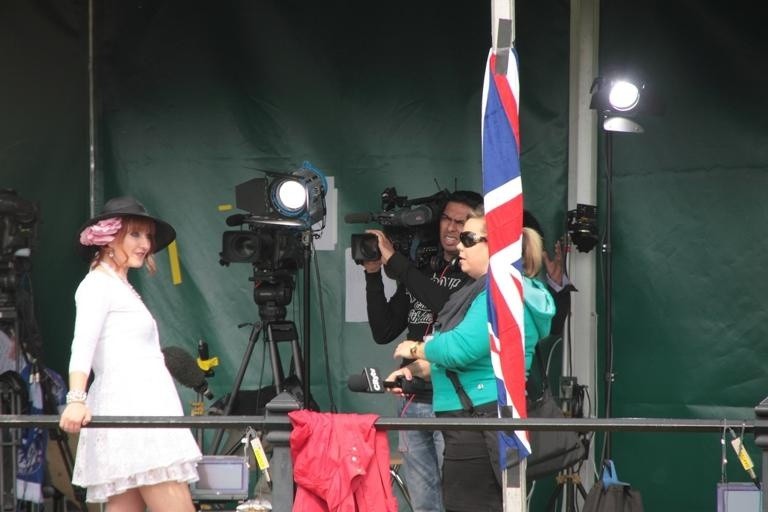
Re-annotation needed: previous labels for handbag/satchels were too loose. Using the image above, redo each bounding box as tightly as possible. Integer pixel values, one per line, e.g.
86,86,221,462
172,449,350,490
583,460,643,512
472,394,586,488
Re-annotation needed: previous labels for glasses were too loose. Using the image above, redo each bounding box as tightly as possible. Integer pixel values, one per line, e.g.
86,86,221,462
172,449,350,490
459,230,487,247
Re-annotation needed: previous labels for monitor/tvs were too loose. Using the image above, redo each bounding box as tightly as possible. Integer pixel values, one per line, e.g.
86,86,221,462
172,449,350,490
716,481,763,512
188,455,249,500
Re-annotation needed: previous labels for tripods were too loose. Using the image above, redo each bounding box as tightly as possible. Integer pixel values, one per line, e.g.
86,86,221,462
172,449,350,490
211,317,320,456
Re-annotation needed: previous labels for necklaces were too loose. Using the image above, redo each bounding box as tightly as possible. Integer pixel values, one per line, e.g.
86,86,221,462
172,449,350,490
117,272,142,300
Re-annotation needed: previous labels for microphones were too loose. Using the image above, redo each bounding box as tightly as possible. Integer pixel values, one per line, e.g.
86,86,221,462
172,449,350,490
347,366,399,397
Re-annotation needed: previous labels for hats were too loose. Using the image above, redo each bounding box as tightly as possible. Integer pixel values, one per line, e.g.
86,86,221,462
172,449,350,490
76,194,176,260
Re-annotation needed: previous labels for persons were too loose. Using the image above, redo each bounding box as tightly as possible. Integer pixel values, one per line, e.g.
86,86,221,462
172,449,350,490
55,194,204,511
511,208,578,512
380,205,555,512
361,188,484,512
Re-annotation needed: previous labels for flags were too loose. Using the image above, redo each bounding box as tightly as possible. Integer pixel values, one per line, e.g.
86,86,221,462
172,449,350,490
475,1,536,474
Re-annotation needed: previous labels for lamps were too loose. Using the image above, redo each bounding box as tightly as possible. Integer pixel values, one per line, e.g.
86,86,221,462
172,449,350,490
592,57,647,117
269,165,329,220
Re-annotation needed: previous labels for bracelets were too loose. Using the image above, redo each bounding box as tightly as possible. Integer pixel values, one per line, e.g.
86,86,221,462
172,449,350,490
409,341,422,359
65,390,87,403
